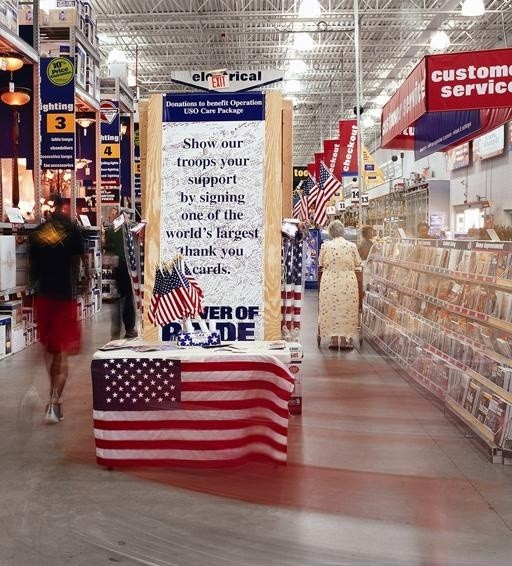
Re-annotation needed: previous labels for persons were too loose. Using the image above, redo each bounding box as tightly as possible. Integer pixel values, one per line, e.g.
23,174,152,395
315,220,363,351
25,194,92,425
357,226,378,260
104,211,139,342
416,222,435,239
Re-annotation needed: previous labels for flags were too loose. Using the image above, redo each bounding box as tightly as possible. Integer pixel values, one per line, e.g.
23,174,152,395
88,356,297,472
297,171,320,223
313,159,343,227
280,230,303,336
292,190,302,218
146,253,204,328
124,220,146,319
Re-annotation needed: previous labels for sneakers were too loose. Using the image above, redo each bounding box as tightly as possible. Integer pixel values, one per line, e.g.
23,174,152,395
43,397,64,425
328,340,339,347
125,330,138,338
340,342,348,348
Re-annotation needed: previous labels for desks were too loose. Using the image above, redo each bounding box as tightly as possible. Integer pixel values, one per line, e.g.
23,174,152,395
91,339,303,471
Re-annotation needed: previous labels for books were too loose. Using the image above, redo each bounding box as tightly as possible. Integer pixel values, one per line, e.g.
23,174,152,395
484,228,500,242
97,337,286,354
361,238,511,455
396,227,406,238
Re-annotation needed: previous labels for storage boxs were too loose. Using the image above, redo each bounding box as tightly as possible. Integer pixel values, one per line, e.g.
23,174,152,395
0,238,102,361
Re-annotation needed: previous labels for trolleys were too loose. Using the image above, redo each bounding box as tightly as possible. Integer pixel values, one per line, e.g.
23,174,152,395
316,264,364,348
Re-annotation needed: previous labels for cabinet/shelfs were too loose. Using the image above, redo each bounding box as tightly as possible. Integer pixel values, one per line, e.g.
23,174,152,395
359,237,512,466
369,180,450,237
